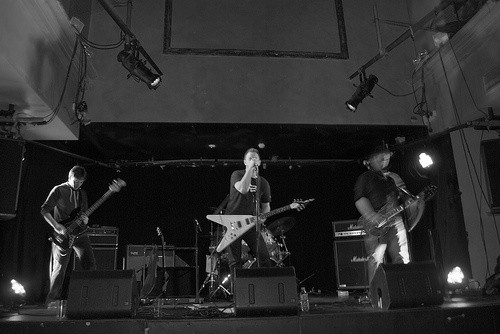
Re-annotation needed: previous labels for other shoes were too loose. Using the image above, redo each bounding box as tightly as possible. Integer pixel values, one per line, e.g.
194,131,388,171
47,301,59,308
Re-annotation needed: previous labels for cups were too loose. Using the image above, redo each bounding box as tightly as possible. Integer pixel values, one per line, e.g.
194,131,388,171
56,299,66,320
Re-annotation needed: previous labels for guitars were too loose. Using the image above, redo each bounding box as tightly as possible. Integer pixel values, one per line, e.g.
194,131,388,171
157,226,168,297
49,179,126,257
363,184,438,238
206,198,315,253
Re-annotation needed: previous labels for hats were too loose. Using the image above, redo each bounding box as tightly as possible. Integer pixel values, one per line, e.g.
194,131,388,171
369,141,392,153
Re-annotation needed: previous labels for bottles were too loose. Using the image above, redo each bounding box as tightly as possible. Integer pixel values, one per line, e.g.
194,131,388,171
299,286,310,312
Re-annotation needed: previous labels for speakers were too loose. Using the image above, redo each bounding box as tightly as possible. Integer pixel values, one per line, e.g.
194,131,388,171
479,137,500,207
73,247,117,270
142,267,197,298
369,263,444,310
233,266,298,316
66,270,138,318
334,239,369,289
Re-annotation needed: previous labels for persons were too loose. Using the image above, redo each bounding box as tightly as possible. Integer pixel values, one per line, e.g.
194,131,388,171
224,148,272,270
41,165,94,308
353,146,425,284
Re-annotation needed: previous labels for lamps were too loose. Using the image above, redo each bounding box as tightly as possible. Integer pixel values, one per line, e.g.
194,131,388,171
117,36,164,91
344,65,379,113
